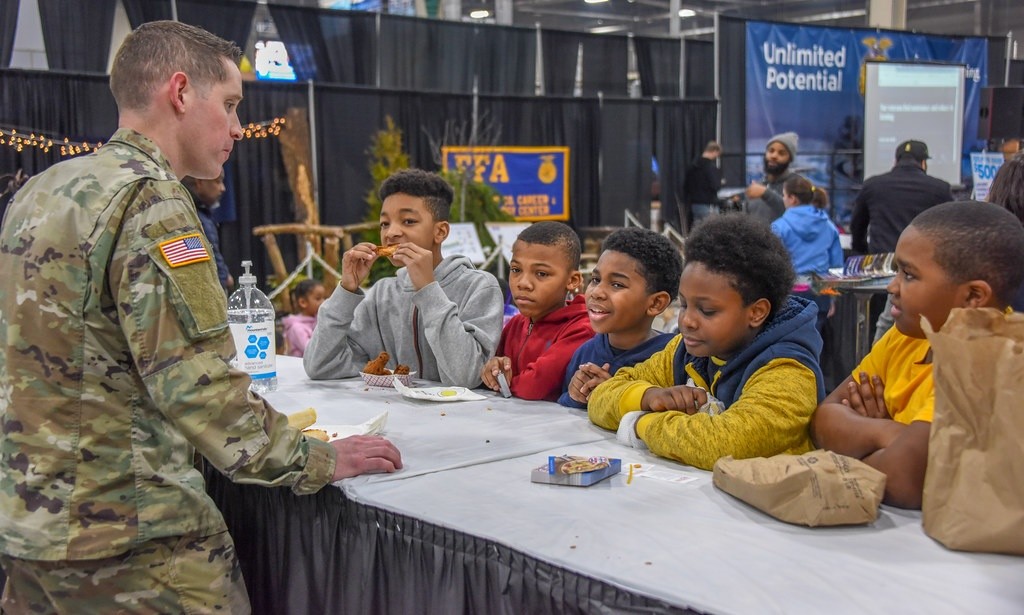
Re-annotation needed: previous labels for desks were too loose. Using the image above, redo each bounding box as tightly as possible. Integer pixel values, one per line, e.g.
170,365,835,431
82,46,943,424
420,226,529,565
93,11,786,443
195,354,1024,615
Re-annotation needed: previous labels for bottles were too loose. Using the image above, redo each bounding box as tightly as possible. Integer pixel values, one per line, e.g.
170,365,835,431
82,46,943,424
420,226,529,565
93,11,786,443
227,262,277,397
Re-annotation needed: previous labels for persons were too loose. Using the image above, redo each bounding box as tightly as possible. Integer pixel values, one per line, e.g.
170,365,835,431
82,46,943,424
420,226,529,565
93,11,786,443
303,169,504,389
770,175,843,334
731,131,799,223
180,166,233,294
282,279,327,357
809,199,1024,510
481,220,595,401
831,116,863,178
0,20,402,615
986,148,1024,227
683,141,723,234
850,138,956,353
588,206,827,471
557,225,684,409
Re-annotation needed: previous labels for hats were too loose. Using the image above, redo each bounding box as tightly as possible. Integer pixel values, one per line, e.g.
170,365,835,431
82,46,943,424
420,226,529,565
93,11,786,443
766,132,800,162
895,140,932,160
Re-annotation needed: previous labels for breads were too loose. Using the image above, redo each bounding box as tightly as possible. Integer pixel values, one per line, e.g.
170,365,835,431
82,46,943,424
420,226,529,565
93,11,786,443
286,406,330,441
